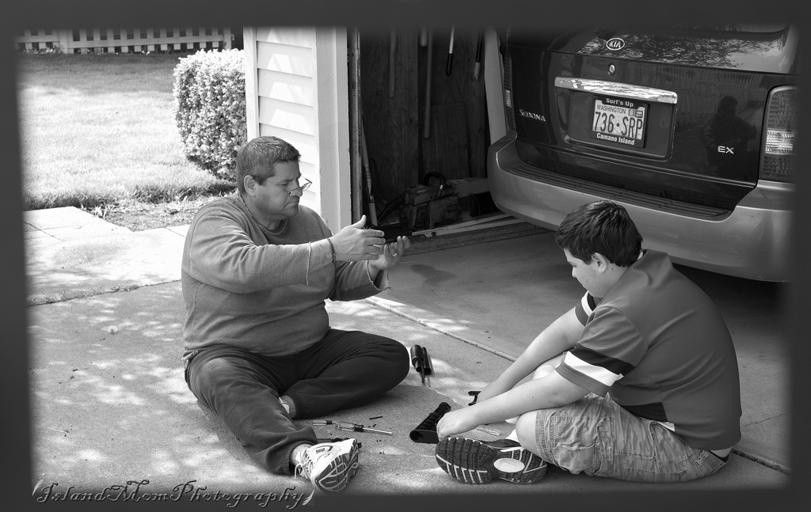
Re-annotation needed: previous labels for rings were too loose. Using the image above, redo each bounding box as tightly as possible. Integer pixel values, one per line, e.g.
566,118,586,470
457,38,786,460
392,252,398,257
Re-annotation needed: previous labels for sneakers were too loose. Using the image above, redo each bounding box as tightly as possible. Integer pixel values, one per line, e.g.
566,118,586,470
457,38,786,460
294,438,358,492
435,437,547,484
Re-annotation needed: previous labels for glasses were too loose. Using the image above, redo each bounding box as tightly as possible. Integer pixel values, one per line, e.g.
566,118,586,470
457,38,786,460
261,174,312,197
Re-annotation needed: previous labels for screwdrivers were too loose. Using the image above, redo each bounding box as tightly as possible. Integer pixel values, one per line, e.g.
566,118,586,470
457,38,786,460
338,422,392,436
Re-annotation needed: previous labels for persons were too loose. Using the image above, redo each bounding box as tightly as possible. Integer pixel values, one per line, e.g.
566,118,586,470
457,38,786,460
180,136,410,498
434,198,742,486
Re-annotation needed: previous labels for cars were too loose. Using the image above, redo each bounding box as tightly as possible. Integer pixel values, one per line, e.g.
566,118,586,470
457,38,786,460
484,9,806,292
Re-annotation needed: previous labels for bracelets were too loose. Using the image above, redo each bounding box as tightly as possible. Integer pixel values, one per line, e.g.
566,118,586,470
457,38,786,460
326,237,336,264
